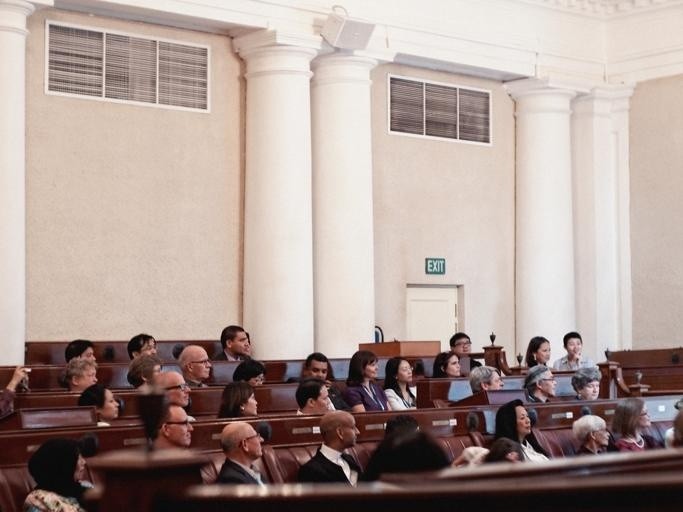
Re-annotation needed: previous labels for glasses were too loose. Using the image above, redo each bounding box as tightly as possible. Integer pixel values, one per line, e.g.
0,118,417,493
238,432,261,449
191,359,211,363
159,419,188,428
166,383,189,392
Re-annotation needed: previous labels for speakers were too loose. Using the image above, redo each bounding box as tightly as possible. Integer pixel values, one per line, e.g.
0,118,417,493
321,12,374,50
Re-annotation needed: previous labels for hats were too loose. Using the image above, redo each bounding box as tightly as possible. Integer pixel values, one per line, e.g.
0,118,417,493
525,364,550,387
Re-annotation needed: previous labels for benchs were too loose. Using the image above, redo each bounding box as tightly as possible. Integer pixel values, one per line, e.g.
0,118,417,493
0,342,682,512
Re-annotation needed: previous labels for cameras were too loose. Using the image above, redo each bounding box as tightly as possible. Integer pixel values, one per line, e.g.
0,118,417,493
18,366,31,372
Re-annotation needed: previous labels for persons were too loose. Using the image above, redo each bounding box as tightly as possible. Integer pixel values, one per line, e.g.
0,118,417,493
1,340,120,426
291,351,448,483
24,439,92,512
433,333,504,393
127,334,211,451
572,398,682,457
524,332,600,403
451,399,549,468
217,325,267,485
